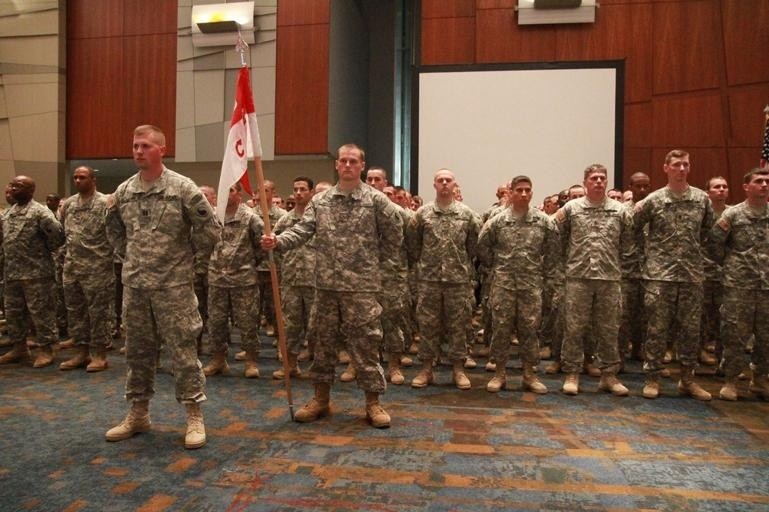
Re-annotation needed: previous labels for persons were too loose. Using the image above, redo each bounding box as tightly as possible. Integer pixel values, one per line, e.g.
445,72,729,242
0,146,768,405
104,124,223,449
257,140,404,430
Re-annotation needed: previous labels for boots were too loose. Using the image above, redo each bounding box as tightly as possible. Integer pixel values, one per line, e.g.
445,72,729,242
294,384,329,421
338,344,507,393
523,344,768,401
1,319,113,371
203,331,311,380
184,403,206,448
365,392,390,428
105,401,151,442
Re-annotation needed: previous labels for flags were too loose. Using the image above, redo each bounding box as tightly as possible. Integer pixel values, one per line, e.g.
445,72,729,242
214,63,263,230
